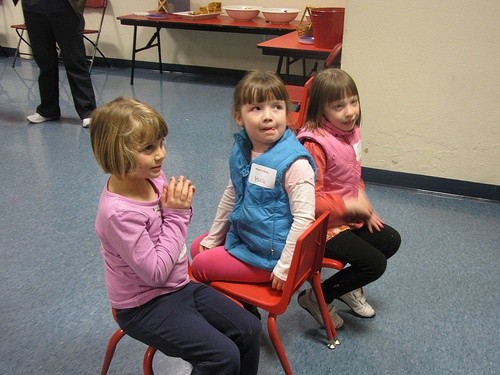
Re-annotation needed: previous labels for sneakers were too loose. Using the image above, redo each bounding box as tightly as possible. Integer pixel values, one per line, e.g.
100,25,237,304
82,117,92,127
296,288,345,330
26,112,54,123
336,288,376,318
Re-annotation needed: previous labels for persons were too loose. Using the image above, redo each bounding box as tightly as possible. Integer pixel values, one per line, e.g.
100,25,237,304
297,68,401,329
91,96,262,375
192,71,317,294
13,0,98,128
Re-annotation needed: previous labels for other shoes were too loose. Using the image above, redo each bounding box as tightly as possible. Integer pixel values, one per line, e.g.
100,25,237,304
244,304,262,321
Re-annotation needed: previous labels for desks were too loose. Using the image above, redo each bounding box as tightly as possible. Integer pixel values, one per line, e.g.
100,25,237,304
116,11,309,85
257,30,334,85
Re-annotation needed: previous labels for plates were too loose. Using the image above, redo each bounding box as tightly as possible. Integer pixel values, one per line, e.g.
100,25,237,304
297,35,315,45
147,13,169,19
171,11,222,20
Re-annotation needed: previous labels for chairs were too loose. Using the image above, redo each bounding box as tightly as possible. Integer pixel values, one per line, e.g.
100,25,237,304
11,25,34,67
285,42,343,136
308,258,346,345
82,0,111,75
211,211,335,375
102,258,244,375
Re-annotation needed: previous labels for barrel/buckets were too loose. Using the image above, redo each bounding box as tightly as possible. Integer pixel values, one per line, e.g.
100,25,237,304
310,7,345,49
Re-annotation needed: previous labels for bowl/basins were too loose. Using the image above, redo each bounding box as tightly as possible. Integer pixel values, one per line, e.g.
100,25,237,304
224,6,261,21
259,8,300,24
